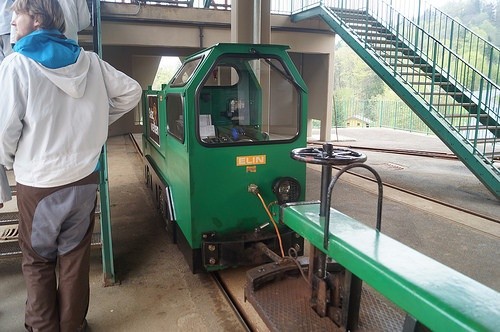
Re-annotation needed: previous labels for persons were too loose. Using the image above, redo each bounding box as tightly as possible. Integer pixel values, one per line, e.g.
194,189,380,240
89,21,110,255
0,0,143,332
